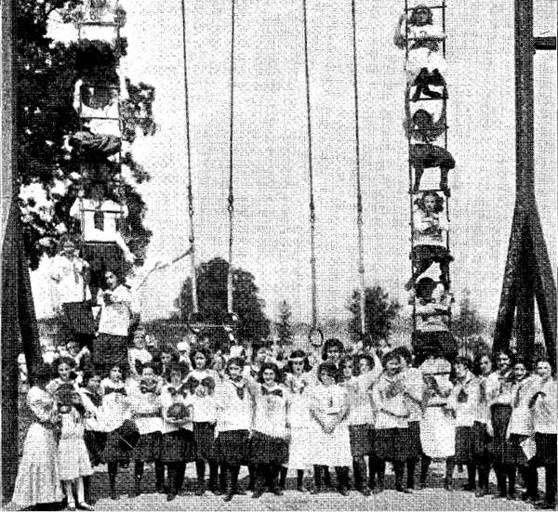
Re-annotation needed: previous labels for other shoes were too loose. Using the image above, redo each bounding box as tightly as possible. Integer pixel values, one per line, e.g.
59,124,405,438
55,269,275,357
443,481,454,490
195,484,205,495
77,500,94,510
224,488,232,501
157,483,166,493
68,501,76,510
234,487,245,495
110,490,119,500
417,480,427,489
208,484,222,495
462,482,554,509
129,490,140,498
167,489,177,501
249,484,413,497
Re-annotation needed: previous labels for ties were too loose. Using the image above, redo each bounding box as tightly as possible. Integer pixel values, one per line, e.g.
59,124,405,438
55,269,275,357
514,386,523,408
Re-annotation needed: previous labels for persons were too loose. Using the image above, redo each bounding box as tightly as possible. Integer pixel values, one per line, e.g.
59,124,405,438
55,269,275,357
395,5,450,99
70,181,139,273
70,64,128,158
91,269,137,371
47,236,102,353
403,109,454,195
12,322,557,510
112,225,139,267
405,198,470,290
407,273,460,359
